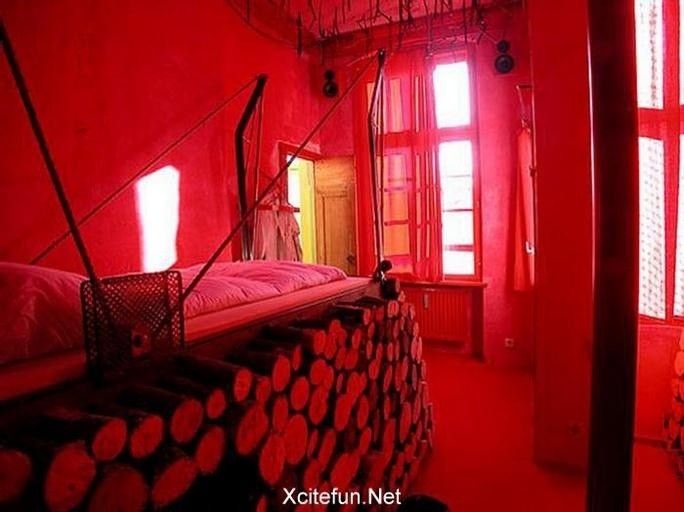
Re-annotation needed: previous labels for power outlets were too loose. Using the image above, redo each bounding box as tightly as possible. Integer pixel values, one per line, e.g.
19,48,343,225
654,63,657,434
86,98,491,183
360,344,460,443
505,338,514,349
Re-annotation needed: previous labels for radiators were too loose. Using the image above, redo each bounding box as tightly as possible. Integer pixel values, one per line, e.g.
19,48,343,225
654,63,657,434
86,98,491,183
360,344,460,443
400,286,473,354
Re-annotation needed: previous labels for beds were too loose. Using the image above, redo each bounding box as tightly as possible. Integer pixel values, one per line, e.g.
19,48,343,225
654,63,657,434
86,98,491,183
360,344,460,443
0,259,372,397
